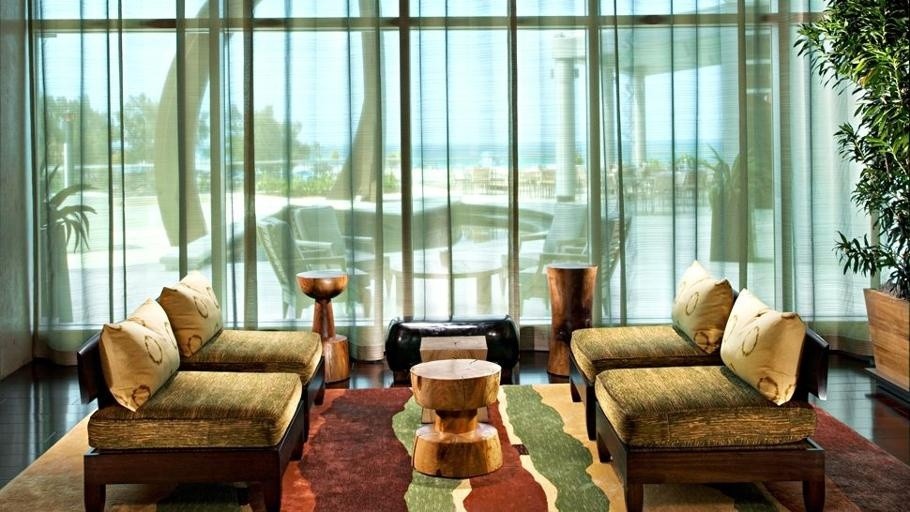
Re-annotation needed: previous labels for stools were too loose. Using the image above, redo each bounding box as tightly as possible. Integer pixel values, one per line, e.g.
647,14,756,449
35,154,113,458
419,335,488,423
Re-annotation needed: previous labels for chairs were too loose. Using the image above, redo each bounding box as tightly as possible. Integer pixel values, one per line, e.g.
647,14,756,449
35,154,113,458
77,321,305,512
258,216,371,319
453,164,708,215
501,203,588,309
520,215,632,315
596,326,830,512
294,206,391,314
156,297,325,442
569,292,739,438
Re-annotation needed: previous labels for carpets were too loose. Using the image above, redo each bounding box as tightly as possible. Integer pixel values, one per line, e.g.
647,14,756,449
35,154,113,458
1,383,909,510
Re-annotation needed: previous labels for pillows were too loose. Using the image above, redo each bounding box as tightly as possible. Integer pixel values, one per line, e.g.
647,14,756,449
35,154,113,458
158,270,222,357
671,260,734,354
99,297,180,412
721,288,807,406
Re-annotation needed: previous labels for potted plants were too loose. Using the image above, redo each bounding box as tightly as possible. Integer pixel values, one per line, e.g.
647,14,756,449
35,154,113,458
39,165,97,323
793,0,909,388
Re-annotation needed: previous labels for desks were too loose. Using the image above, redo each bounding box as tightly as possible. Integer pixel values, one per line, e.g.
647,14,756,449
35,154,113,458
410,358,502,478
389,251,509,315
